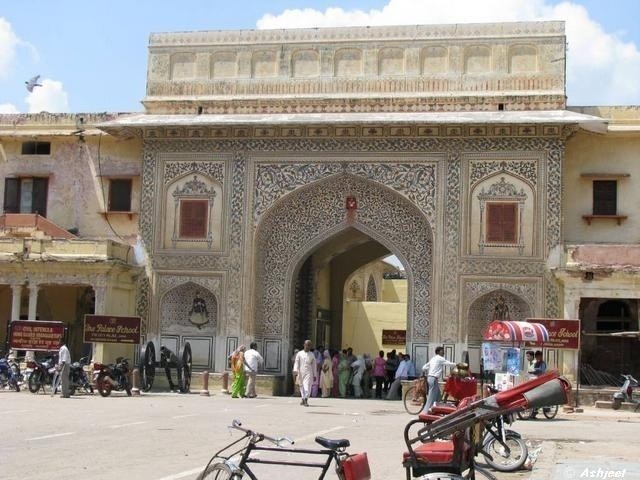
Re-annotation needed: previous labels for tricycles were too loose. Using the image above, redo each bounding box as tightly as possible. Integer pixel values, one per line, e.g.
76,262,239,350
196,368,572,480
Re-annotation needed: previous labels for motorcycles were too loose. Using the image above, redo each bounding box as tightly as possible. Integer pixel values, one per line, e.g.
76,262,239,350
428,401,527,472
0,352,133,397
612,375,640,410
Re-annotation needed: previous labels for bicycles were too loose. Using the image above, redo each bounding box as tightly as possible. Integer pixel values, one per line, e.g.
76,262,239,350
517,404,558,419
404,370,460,416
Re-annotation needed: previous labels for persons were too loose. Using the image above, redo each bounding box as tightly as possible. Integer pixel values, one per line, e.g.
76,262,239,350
528,351,546,376
420,347,461,416
244,343,264,398
526,351,537,374
59,344,72,398
230,344,254,398
291,339,415,406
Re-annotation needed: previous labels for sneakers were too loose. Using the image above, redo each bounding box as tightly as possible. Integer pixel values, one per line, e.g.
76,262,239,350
232,394,257,397
300,398,309,406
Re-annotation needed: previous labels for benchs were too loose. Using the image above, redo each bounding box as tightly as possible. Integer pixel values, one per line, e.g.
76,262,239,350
402,394,494,480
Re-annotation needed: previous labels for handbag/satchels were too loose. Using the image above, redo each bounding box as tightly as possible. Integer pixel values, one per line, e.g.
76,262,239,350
417,377,428,394
